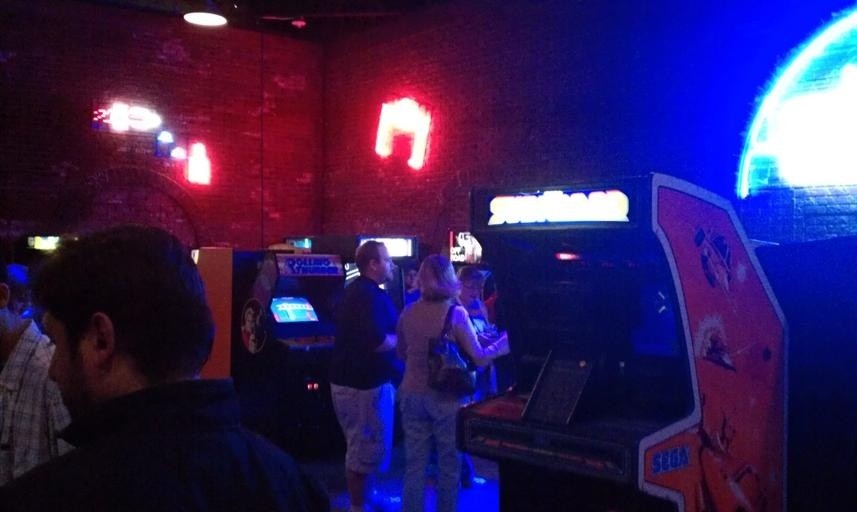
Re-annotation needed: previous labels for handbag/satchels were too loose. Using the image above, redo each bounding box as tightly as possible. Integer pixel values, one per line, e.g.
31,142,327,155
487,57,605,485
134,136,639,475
424,301,480,398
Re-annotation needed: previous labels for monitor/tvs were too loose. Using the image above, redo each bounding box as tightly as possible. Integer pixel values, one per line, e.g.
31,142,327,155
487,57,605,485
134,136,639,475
270,297,319,323
